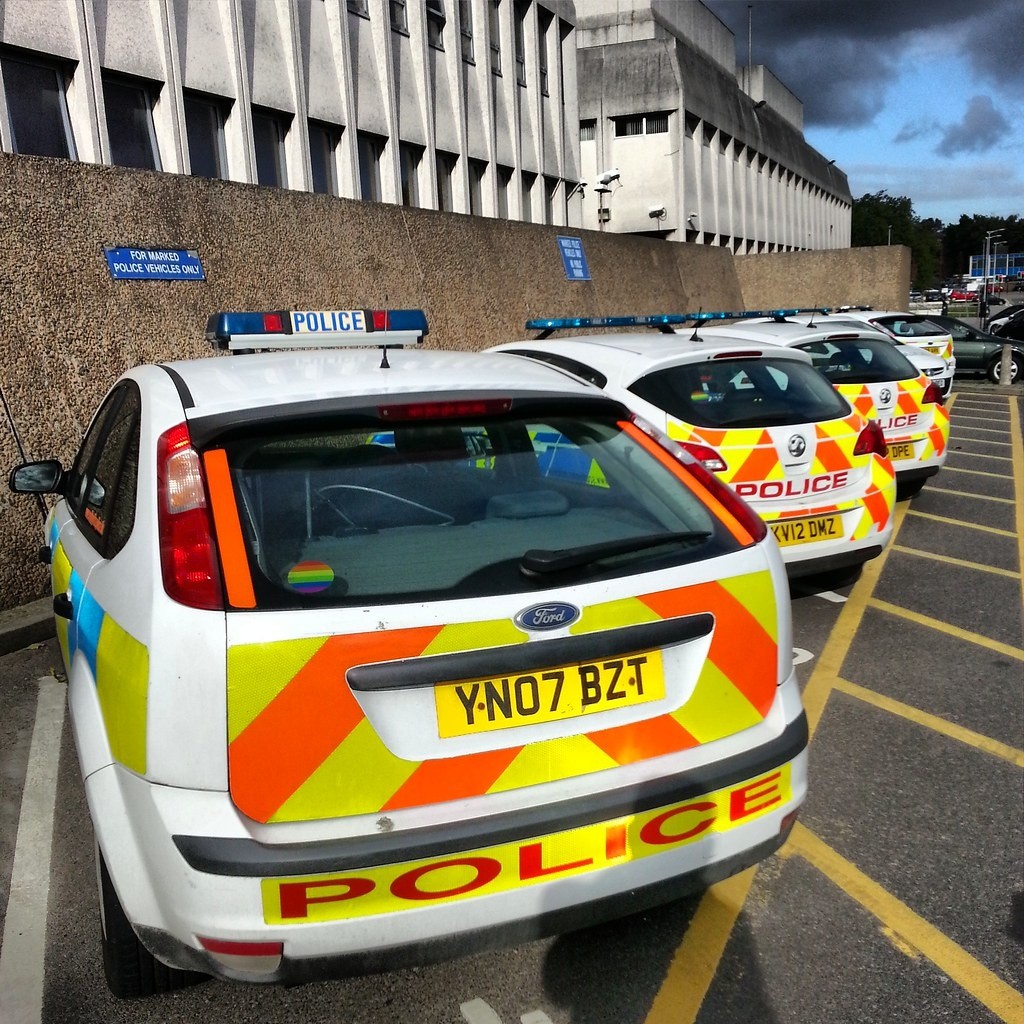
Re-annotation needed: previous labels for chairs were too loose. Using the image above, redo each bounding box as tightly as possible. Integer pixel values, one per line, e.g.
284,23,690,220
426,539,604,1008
484,489,572,520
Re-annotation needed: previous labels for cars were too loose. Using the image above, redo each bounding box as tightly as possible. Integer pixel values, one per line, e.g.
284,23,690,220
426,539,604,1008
479,317,898,585
8,311,810,998
692,305,951,497
917,313,1023,385
730,307,952,405
910,274,1023,342
825,304,956,376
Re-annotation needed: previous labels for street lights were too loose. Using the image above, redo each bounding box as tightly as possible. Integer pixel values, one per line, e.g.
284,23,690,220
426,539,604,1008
986,227,1005,279
888,224,892,246
991,240,1007,295
982,234,1002,301
